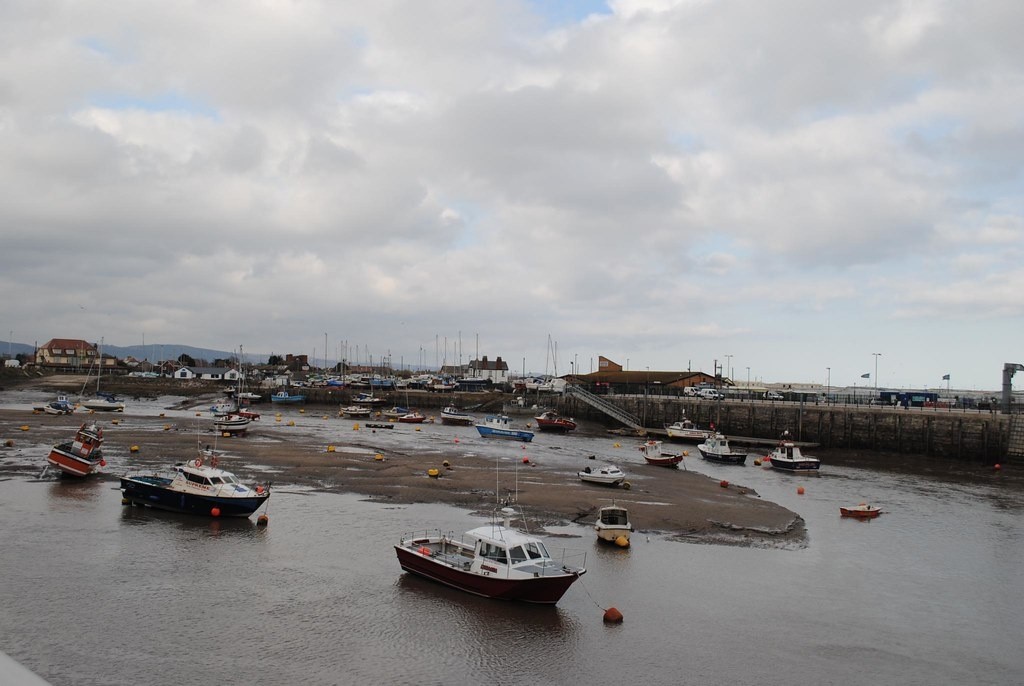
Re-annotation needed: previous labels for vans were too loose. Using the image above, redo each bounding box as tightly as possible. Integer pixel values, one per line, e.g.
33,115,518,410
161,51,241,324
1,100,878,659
683,386,701,397
701,389,725,400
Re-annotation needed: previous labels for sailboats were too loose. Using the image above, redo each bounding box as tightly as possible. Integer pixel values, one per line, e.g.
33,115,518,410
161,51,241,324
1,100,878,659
74,336,124,411
210,345,262,431
514,335,571,393
306,331,487,390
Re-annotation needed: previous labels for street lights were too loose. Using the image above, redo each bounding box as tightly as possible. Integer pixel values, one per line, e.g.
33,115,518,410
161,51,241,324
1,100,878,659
872,352,882,402
724,354,733,388
825,367,831,406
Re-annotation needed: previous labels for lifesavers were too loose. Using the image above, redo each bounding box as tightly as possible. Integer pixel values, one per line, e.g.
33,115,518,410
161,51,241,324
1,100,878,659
648,441,655,445
210,455,218,467
194,458,202,467
418,546,429,556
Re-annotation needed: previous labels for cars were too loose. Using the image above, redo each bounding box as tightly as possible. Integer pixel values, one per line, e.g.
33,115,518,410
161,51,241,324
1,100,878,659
762,391,784,400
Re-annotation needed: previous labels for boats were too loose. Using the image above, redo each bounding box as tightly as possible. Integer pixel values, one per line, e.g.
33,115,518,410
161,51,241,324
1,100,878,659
382,391,427,422
341,405,375,417
269,384,308,403
45,420,106,477
534,411,577,433
768,429,821,473
638,438,689,466
840,503,883,518
440,405,478,424
31,393,77,414
578,465,626,487
475,413,535,443
697,430,747,465
119,418,272,517
394,457,587,606
350,392,389,407
595,497,632,547
